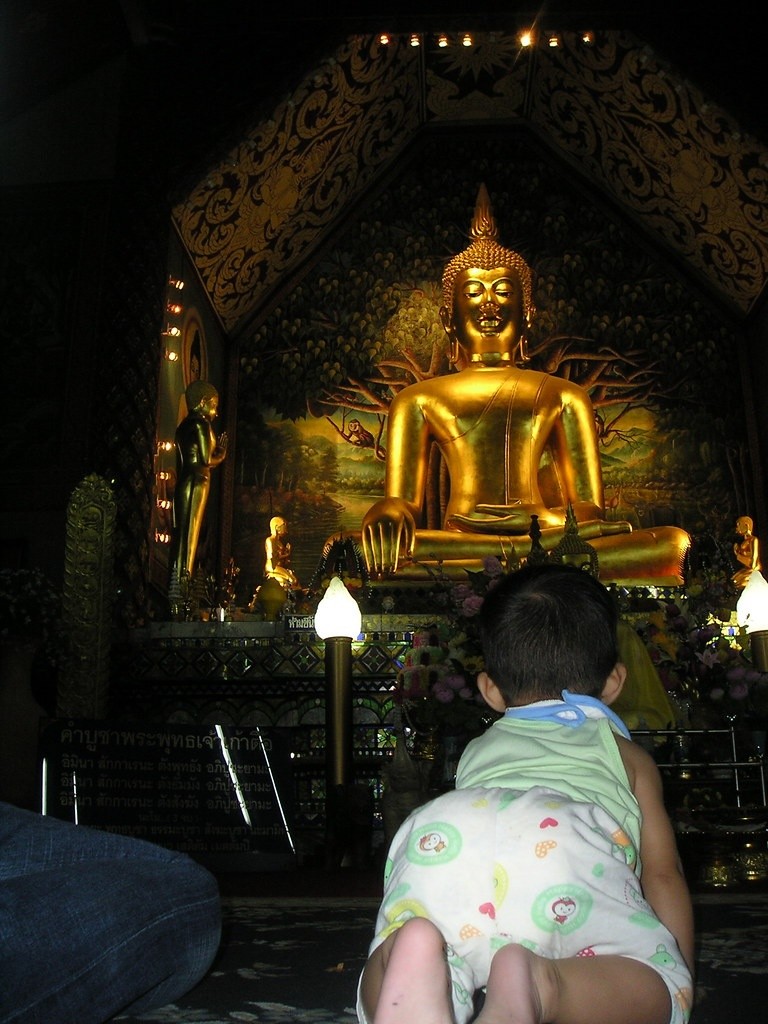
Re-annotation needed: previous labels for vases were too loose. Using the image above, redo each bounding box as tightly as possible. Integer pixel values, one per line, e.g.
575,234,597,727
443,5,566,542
688,700,748,730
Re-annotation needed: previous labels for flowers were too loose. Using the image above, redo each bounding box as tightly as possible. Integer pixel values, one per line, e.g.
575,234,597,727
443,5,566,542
400,567,768,739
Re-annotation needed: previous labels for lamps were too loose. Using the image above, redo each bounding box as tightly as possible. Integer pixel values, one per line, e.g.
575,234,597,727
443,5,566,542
727,568,768,672
313,572,367,880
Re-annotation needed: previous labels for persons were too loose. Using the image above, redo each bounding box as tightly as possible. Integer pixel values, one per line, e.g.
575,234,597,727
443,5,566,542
321,181,693,587
165,380,228,602
548,526,676,733
347,561,695,1024
262,516,297,585
1,799,222,1024
730,515,763,587
